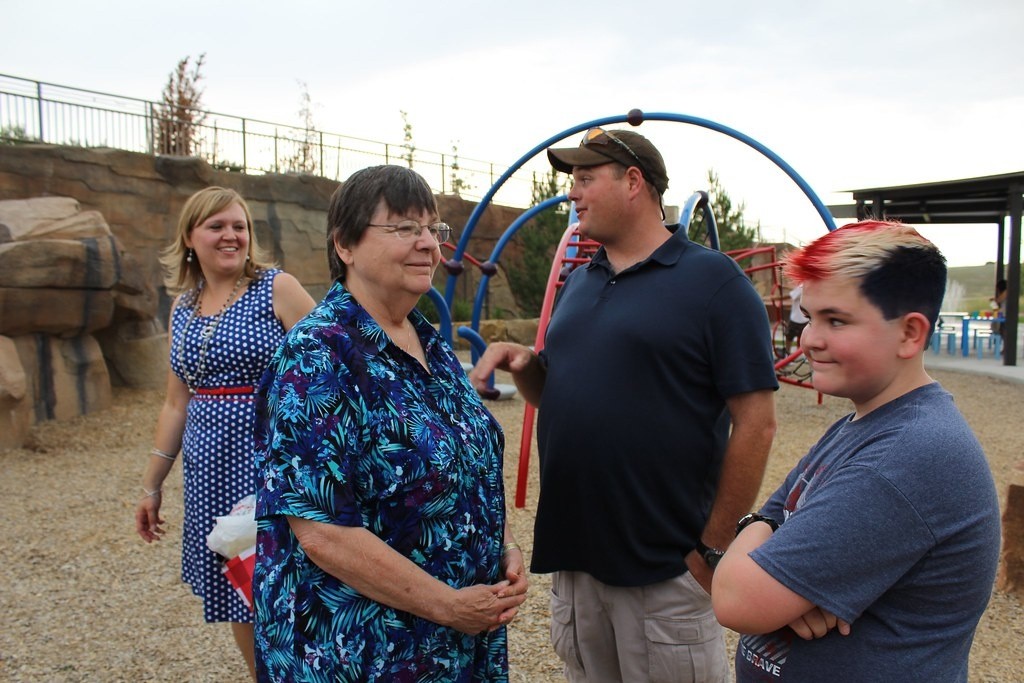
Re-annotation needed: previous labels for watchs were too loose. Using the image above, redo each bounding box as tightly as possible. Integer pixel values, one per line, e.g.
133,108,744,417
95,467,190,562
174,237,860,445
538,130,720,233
735,513,779,533
696,538,726,569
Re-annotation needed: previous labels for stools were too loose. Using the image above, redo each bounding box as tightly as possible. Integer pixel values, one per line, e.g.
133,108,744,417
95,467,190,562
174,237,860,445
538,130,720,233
930,326,1001,359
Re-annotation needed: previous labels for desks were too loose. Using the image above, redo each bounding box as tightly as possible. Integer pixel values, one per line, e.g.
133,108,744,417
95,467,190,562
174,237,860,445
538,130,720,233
939,312,969,329
961,317,1004,356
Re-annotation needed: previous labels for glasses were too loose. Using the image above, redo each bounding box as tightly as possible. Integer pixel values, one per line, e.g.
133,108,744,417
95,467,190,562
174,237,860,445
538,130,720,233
365,220,452,245
579,125,648,174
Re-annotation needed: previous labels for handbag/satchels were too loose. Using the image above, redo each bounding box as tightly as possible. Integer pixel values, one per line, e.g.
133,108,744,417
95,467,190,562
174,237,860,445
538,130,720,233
222,546,259,612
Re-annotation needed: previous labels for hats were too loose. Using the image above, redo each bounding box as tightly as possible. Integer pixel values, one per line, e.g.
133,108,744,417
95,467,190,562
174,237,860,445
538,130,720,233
547,130,668,193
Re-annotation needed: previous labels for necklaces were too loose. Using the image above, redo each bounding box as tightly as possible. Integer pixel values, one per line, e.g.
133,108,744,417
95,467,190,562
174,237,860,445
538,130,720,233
180,277,244,394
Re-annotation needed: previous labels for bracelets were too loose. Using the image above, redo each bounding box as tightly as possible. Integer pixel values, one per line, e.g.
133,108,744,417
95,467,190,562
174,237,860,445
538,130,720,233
502,543,521,554
150,447,176,461
138,487,160,500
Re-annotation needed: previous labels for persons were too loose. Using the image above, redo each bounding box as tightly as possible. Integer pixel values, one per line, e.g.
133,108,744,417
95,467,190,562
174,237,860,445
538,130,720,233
990,280,1007,355
137,187,319,677
254,166,527,683
770,282,810,362
471,131,780,683
712,220,1000,683
